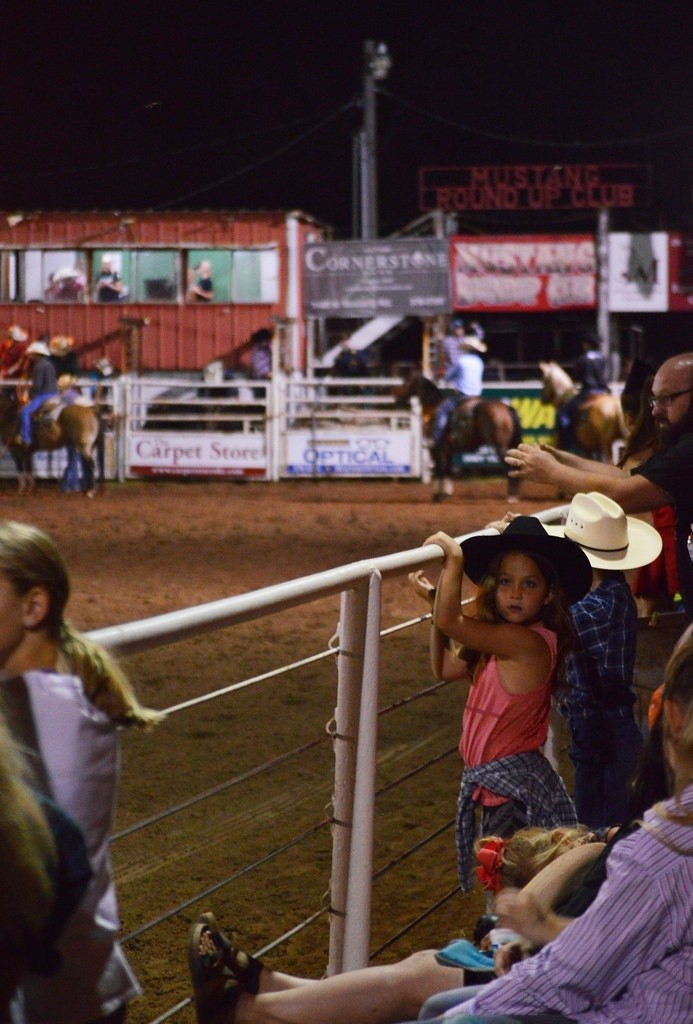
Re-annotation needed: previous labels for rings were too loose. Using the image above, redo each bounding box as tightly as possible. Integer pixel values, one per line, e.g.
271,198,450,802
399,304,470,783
518,460,523,466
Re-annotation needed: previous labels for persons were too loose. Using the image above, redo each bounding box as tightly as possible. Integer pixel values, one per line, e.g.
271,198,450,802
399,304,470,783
557,333,610,433
19,341,57,445
44,267,85,303
190,623,693,1024
504,353,693,623
428,320,488,447
188,260,213,300
58,374,82,492
328,334,368,410
95,253,122,301
485,491,663,826
0,324,28,379
0,520,166,1024
250,326,272,378
406,516,593,913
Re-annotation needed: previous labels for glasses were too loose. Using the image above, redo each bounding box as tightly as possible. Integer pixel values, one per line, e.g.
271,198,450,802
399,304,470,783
645,386,690,406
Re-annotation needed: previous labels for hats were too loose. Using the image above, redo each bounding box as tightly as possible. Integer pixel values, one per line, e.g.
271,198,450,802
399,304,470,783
6,326,29,343
251,327,276,343
27,339,52,359
463,335,488,354
542,490,663,570
462,515,594,609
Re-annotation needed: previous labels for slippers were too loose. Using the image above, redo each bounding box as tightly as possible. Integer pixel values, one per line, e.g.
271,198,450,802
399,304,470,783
186,920,242,1023
204,912,262,995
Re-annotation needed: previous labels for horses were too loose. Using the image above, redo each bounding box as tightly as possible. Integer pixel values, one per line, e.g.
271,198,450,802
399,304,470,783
538,362,631,465
0,383,118,498
391,362,524,501
